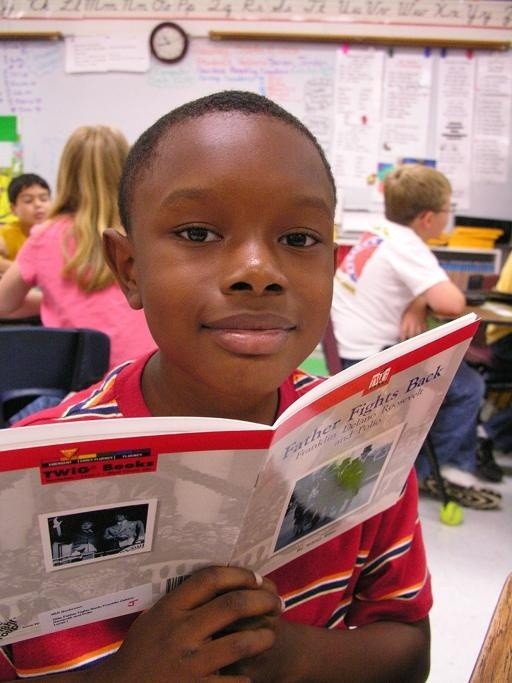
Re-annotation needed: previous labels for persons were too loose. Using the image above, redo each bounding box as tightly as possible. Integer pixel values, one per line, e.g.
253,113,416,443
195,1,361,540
1,122,161,426
1,173,53,274
1,89,435,682
330,163,502,511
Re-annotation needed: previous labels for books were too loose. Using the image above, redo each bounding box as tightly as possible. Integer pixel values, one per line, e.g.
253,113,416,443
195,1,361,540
1,309,482,647
429,227,512,425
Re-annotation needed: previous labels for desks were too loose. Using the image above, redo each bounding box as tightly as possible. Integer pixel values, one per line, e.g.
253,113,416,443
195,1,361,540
431,279,511,369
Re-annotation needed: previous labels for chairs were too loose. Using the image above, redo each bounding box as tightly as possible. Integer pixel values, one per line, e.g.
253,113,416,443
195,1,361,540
0,324,116,437
317,304,466,536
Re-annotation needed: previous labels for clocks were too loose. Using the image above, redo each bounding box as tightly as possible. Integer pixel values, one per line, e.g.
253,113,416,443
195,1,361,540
147,18,190,65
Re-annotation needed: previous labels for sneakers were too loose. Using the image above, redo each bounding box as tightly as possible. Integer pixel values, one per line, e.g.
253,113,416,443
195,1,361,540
423,463,502,509
474,436,502,482
